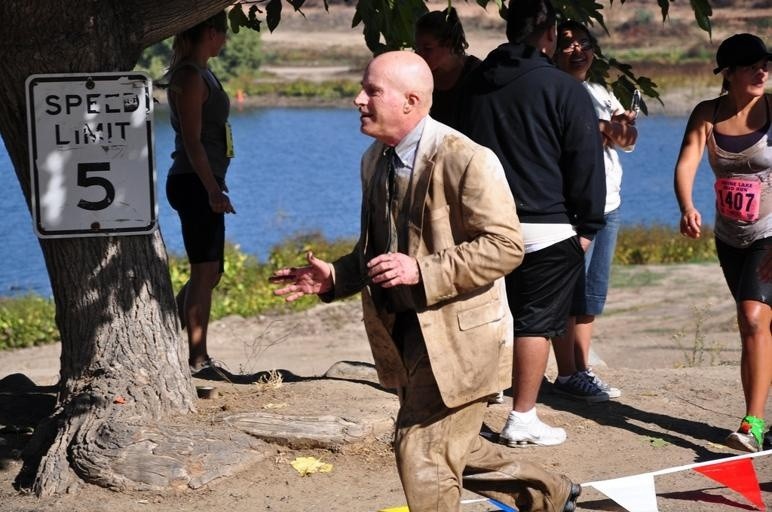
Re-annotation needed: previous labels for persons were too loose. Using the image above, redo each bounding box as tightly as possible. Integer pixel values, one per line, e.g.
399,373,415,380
754,244,772,284
673,32,772,456
415,3,638,450
263,50,584,512
167,6,239,383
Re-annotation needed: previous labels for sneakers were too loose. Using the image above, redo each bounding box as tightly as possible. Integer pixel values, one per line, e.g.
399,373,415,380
580,367,621,398
499,413,567,448
551,372,609,404
725,415,765,452
188,356,233,381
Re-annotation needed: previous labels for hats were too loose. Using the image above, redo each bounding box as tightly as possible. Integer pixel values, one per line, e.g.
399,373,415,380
712,34,772,75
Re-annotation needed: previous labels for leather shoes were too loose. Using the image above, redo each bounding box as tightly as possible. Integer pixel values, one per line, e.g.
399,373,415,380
564,483,581,512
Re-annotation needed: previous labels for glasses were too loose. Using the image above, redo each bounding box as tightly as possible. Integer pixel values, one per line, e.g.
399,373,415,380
559,39,592,54
